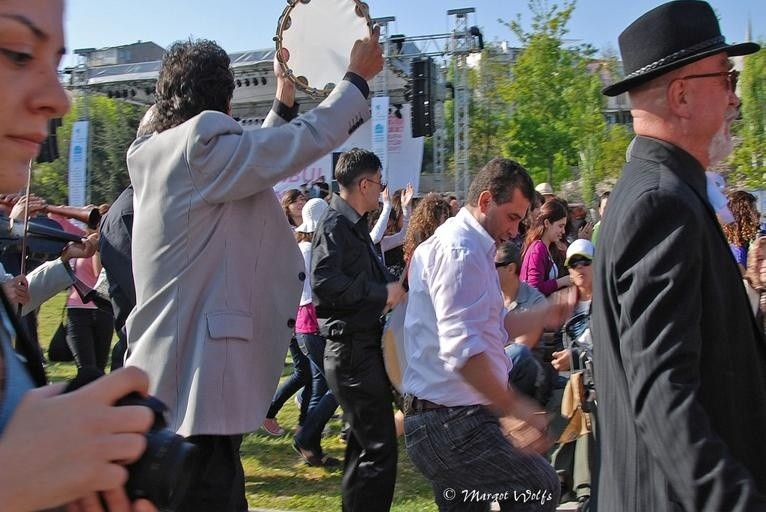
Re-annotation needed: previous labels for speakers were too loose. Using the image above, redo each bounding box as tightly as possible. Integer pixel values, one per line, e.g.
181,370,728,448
38,105,63,163
407,58,436,138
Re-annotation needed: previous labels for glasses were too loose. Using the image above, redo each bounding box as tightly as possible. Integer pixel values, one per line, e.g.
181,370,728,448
567,256,589,265
358,175,389,192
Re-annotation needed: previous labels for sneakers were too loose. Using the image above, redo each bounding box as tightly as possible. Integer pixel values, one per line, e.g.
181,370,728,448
265,415,284,436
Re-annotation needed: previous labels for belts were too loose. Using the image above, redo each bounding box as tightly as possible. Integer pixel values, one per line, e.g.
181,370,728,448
407,395,443,413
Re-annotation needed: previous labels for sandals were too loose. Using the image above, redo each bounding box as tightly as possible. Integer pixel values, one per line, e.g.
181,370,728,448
292,439,342,468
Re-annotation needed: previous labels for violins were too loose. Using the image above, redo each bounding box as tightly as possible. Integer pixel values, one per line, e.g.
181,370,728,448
0,215,100,262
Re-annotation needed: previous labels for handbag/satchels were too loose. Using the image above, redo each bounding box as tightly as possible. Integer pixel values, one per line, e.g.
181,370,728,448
46,319,76,363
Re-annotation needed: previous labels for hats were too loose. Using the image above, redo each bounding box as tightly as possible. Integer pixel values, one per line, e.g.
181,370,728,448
563,238,596,267
601,0,762,96
296,197,330,236
534,181,555,197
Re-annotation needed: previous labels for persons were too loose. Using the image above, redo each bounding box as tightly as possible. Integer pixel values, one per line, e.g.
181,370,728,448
122,26,383,512
590,0,766,511
0,1,167,510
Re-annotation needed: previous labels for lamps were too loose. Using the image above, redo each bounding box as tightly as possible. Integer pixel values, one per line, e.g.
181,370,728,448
228,113,267,127
390,102,404,119
106,68,270,99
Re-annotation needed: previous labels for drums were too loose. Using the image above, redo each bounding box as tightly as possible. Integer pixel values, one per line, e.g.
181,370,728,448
273,0,373,99
381,288,408,400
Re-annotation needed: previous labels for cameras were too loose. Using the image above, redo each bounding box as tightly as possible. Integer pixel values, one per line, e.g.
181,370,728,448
54,361,198,511
